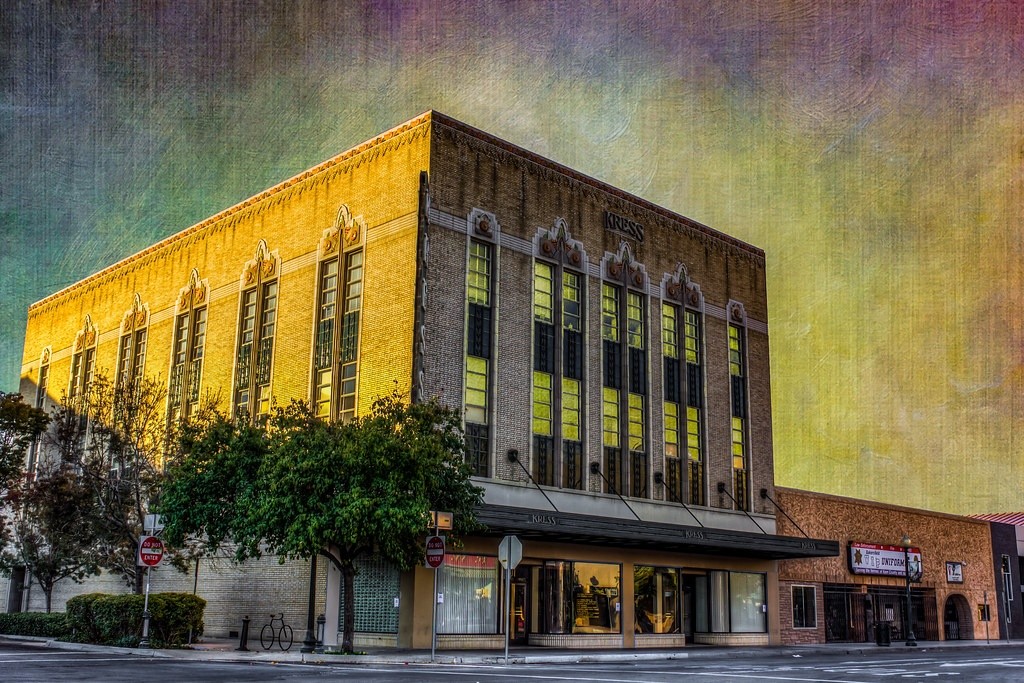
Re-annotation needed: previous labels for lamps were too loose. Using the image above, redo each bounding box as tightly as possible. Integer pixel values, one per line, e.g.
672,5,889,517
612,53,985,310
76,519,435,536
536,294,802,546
315,614,326,652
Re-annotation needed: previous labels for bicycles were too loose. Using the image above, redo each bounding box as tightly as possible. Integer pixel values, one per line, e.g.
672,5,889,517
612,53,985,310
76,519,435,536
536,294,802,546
260,612,294,651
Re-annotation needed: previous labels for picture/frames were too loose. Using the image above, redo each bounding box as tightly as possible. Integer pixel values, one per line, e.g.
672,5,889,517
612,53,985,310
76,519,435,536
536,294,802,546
946,561,963,584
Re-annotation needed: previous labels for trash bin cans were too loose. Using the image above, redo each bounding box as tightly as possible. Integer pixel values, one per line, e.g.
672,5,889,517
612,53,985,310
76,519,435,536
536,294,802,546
874,620,893,647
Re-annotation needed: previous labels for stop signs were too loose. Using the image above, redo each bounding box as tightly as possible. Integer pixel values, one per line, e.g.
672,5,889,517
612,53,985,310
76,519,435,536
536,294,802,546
425,536,445,569
138,536,165,567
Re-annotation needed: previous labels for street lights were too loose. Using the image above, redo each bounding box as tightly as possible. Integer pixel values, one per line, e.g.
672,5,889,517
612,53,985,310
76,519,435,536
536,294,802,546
900,532,917,648
